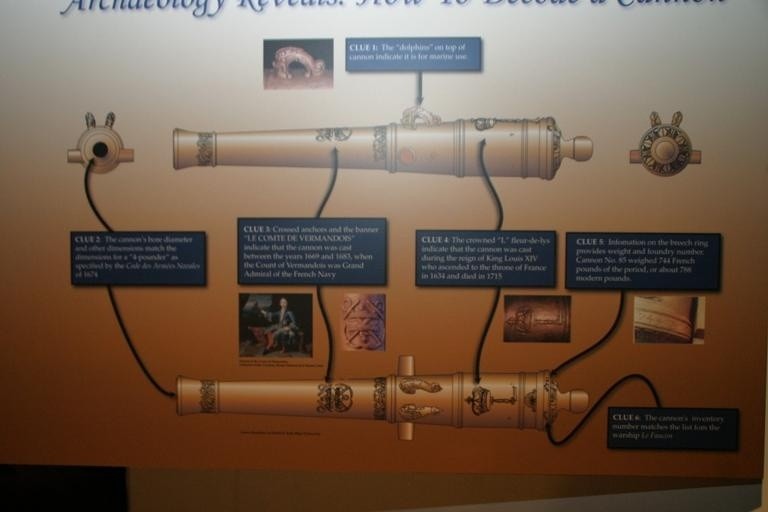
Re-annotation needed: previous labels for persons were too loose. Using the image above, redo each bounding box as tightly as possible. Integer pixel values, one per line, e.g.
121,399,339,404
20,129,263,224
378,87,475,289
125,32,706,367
258,297,297,356
